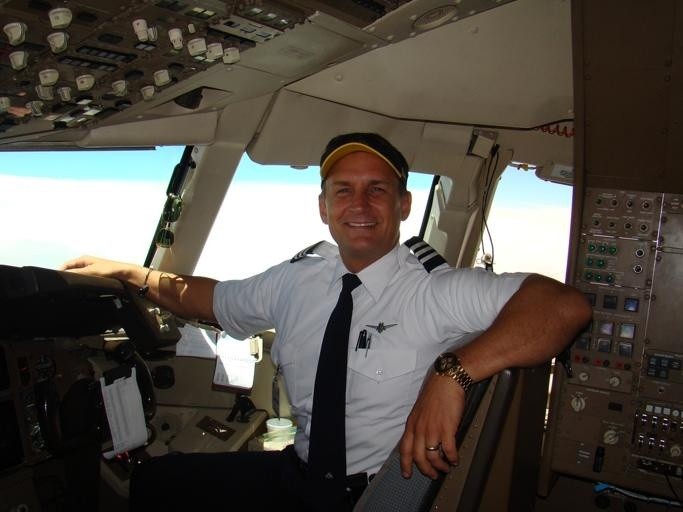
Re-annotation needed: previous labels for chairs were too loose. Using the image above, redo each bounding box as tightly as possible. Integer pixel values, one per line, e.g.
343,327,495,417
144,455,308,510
349,359,516,511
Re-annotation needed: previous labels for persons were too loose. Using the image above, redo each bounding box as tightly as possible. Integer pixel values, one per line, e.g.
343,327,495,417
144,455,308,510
58,129,596,509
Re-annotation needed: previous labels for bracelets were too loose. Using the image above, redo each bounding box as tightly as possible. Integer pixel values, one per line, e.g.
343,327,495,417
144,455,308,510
141,266,155,291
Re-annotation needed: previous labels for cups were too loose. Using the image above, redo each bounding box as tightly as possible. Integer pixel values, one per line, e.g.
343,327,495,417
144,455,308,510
265,417,293,436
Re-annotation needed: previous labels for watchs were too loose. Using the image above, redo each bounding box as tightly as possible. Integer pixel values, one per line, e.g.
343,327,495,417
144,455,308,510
432,351,473,393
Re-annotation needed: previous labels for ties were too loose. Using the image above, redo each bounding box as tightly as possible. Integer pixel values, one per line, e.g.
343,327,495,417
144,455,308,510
308,274,362,479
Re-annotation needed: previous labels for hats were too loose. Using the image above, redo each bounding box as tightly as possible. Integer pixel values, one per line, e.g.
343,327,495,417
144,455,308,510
320,133,409,191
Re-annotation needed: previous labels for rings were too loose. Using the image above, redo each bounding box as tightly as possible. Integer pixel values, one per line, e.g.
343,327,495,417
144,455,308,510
424,446,438,452
